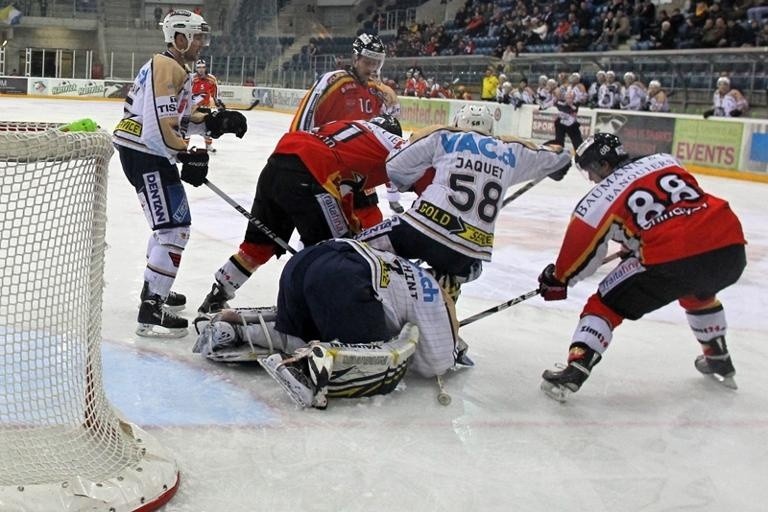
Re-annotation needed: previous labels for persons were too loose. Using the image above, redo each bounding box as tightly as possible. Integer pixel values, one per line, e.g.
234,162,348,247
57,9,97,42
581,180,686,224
9,68,19,76
108,4,247,331
188,56,221,156
353,102,572,281
381,0,768,118
533,137,749,397
193,232,474,410
199,113,435,315
289,34,398,228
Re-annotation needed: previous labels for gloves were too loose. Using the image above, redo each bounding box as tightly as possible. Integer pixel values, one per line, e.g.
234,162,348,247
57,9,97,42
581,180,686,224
539,264,567,301
205,110,247,139
180,148,209,185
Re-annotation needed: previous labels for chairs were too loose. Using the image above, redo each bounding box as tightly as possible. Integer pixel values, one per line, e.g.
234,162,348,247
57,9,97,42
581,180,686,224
365,1,768,115
1,2,359,95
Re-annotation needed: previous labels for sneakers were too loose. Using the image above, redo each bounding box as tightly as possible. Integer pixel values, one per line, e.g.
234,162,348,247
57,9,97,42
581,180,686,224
542,346,601,391
695,340,735,377
138,281,240,346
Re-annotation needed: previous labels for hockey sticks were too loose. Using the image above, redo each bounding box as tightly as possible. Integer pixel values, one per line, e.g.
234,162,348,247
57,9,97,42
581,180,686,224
199,100,259,111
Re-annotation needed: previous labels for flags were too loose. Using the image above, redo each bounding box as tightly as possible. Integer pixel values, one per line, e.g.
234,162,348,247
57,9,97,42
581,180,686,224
0,6,25,24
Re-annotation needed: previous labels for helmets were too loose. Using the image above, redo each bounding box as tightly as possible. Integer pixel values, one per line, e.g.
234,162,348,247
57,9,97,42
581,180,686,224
163,9,213,52
454,102,494,135
574,134,629,168
498,70,662,93
350,33,385,78
370,113,402,138
195,58,207,74
